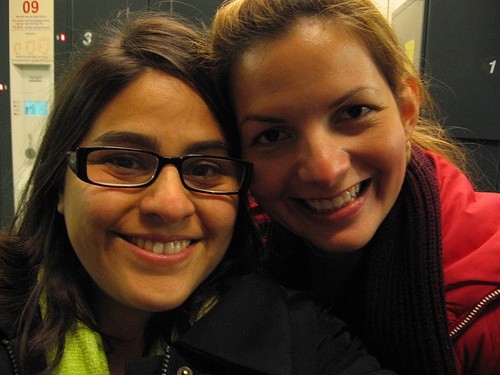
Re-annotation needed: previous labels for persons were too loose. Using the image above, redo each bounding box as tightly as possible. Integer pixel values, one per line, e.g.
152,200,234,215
205,0,499,373
0,11,379,373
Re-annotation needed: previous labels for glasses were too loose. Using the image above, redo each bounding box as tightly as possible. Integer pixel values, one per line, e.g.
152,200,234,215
66,146,255,194
